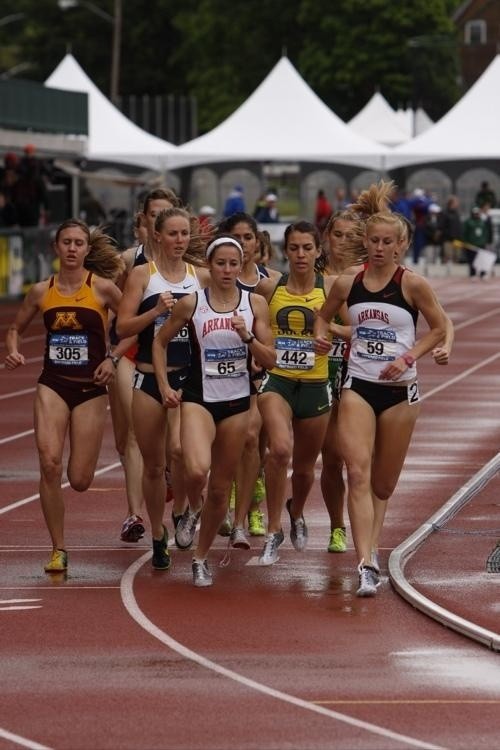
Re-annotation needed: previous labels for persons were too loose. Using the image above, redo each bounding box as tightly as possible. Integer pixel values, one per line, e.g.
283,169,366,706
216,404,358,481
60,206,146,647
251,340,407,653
0,142,500,598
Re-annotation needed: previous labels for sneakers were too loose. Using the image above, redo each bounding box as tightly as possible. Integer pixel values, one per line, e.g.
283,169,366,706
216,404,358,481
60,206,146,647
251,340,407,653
44,549,67,571
122,481,380,596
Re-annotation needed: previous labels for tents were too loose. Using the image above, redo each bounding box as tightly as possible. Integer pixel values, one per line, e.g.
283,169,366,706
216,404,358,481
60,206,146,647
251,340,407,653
4,48,499,216
